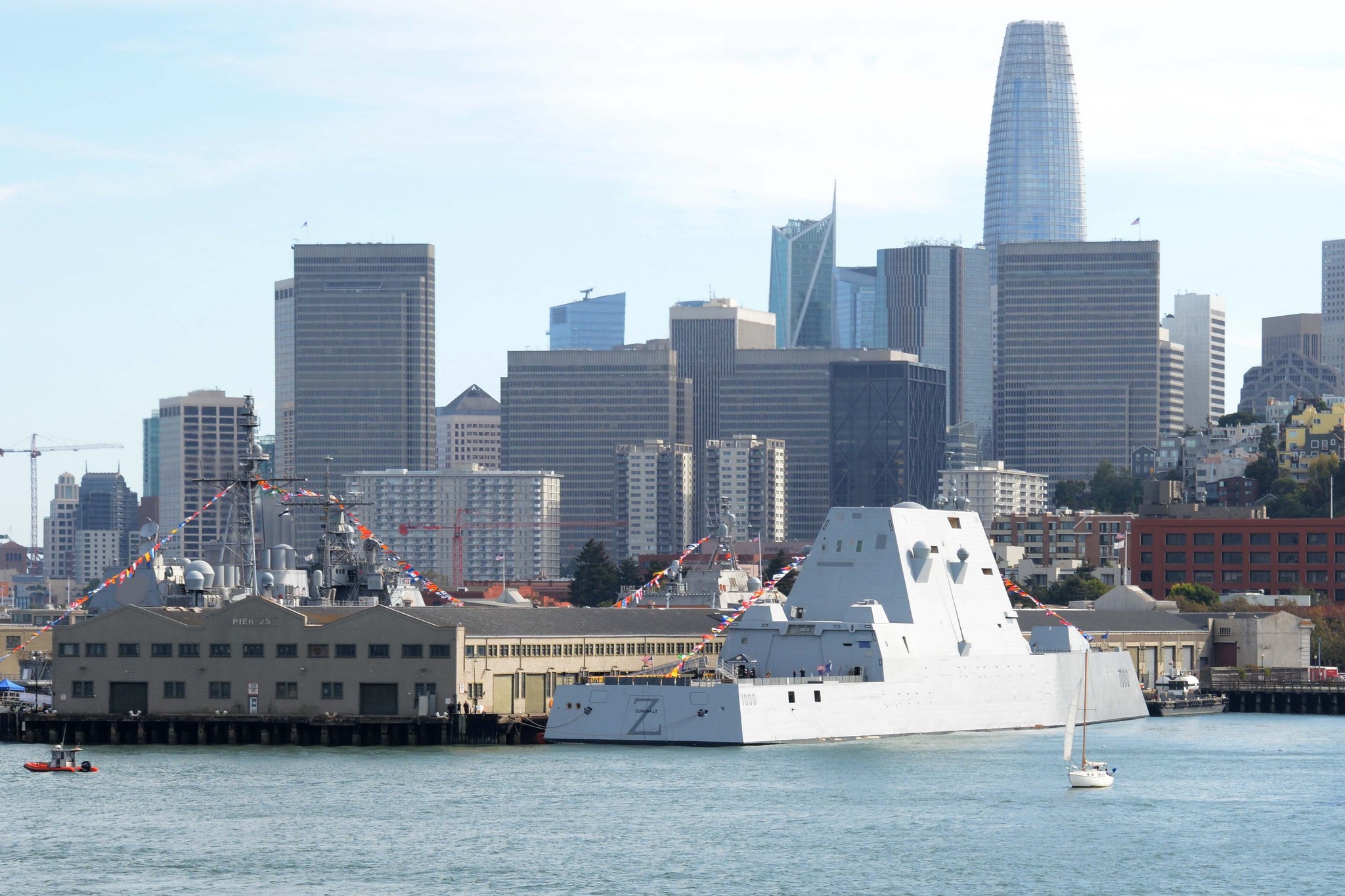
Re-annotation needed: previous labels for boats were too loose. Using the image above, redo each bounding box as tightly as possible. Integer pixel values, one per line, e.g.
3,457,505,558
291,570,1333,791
543,500,1151,744
1145,692,1230,715
24,722,99,772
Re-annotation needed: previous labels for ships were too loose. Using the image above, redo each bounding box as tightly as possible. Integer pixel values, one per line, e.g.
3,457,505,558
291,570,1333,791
84,395,464,610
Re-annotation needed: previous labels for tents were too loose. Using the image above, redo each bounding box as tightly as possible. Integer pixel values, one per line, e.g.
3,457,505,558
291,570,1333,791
0,678,25,693
724,653,760,678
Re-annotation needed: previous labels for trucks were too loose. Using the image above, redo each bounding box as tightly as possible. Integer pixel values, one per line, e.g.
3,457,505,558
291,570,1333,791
1310,666,1344,683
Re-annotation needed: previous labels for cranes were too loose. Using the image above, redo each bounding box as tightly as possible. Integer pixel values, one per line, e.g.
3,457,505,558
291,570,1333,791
0,433,124,577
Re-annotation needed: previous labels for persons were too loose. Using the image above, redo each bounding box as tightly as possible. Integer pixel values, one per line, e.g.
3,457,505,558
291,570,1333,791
449,701,454,713
463,701,469,713
742,666,806,678
1154,689,1158,701
1105,769,1110,776
1095,766,1099,770
66,751,72,762
8,703,58,714
457,702,461,714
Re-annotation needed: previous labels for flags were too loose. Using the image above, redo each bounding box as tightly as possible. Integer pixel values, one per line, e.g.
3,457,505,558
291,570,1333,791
496,552,504,560
817,662,832,676
640,655,652,665
1102,633,1107,639
1114,540,1124,549
753,537,759,542
1115,531,1125,539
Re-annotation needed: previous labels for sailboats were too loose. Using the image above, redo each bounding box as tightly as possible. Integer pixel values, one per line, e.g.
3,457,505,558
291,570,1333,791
1063,650,1117,787
